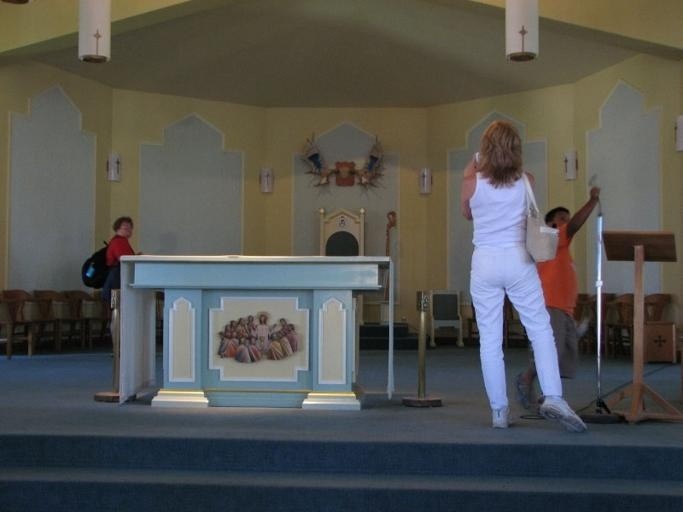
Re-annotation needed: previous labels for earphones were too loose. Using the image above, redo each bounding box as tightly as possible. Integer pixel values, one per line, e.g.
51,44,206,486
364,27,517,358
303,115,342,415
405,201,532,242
552,221,556,228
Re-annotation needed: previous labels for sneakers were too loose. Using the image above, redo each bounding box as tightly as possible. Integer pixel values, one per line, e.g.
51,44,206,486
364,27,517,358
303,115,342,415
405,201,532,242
540,397,588,433
512,372,531,410
492,405,519,429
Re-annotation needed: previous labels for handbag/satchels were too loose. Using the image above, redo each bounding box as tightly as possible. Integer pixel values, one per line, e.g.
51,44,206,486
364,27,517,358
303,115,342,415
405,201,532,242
521,168,560,261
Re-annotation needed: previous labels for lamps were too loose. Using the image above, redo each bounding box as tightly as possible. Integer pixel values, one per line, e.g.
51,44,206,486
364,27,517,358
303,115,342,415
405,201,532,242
105,152,123,182
505,0,538,62
77,0,112,63
418,168,432,194
561,151,577,180
258,167,273,193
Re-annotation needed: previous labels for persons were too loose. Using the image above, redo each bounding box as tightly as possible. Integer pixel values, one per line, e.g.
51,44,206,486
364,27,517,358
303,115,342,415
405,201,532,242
217,311,299,363
513,187,601,410
104,216,143,357
459,120,588,435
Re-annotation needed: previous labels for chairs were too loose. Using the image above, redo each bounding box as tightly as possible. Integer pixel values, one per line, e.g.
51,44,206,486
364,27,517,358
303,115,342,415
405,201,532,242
0,289,110,363
467,293,670,360
428,289,463,348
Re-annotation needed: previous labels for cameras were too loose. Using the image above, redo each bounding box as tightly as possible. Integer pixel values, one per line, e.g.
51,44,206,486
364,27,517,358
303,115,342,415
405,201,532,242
476,152,481,163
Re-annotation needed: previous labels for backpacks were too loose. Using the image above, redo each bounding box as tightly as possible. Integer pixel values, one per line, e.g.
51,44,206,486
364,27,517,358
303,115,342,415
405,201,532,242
82,244,107,289
